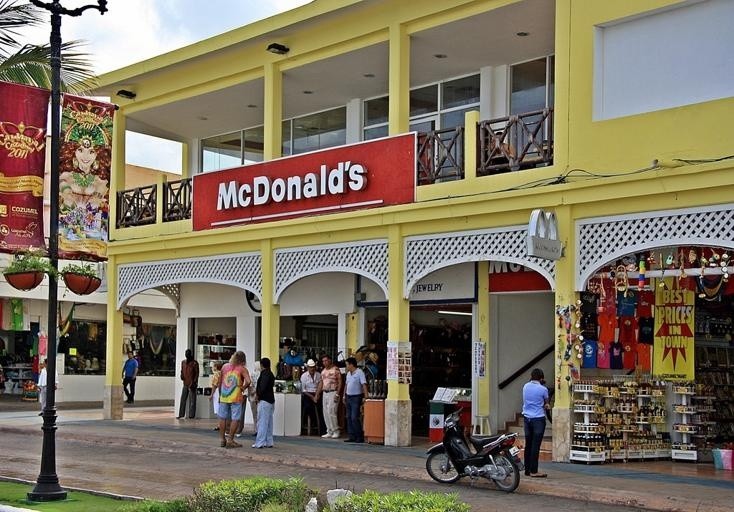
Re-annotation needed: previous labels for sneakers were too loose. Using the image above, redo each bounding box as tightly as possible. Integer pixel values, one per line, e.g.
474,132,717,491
251,443,263,448
524,472,530,476
353,438,364,443
219,440,226,447
331,433,341,439
233,432,242,438
263,444,274,448
211,426,220,431
344,438,355,442
224,431,231,437
321,433,332,438
530,472,548,478
225,441,243,449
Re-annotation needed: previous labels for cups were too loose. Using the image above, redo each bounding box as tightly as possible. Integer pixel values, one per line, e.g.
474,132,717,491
272,386,276,392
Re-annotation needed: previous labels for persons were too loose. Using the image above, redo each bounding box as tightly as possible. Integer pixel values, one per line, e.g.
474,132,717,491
208,350,275,449
284,342,381,443
176,349,200,419
122,351,139,404
38,358,58,416
521,368,552,475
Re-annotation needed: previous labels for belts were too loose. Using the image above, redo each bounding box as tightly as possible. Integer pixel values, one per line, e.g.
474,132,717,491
323,389,336,393
345,393,360,399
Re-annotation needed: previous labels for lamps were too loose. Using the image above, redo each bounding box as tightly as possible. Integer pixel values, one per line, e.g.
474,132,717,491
115,89,138,100
264,42,291,57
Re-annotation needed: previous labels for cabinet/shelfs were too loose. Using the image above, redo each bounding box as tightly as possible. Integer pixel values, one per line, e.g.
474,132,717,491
194,343,237,420
0,363,35,398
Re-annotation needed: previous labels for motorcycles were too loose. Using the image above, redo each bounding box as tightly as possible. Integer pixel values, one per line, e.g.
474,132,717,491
425,406,525,494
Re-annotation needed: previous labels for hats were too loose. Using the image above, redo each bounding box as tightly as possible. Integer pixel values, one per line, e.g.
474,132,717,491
303,358,318,368
367,351,379,365
355,351,368,363
366,364,379,380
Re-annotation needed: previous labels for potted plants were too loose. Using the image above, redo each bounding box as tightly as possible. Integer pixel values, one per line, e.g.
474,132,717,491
57,258,104,301
2,247,59,294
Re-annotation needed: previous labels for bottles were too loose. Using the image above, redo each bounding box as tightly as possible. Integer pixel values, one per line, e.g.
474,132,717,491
605,435,622,449
573,422,598,431
574,399,600,411
614,396,636,412
574,379,618,395
638,407,666,423
572,434,602,446
596,408,621,422
